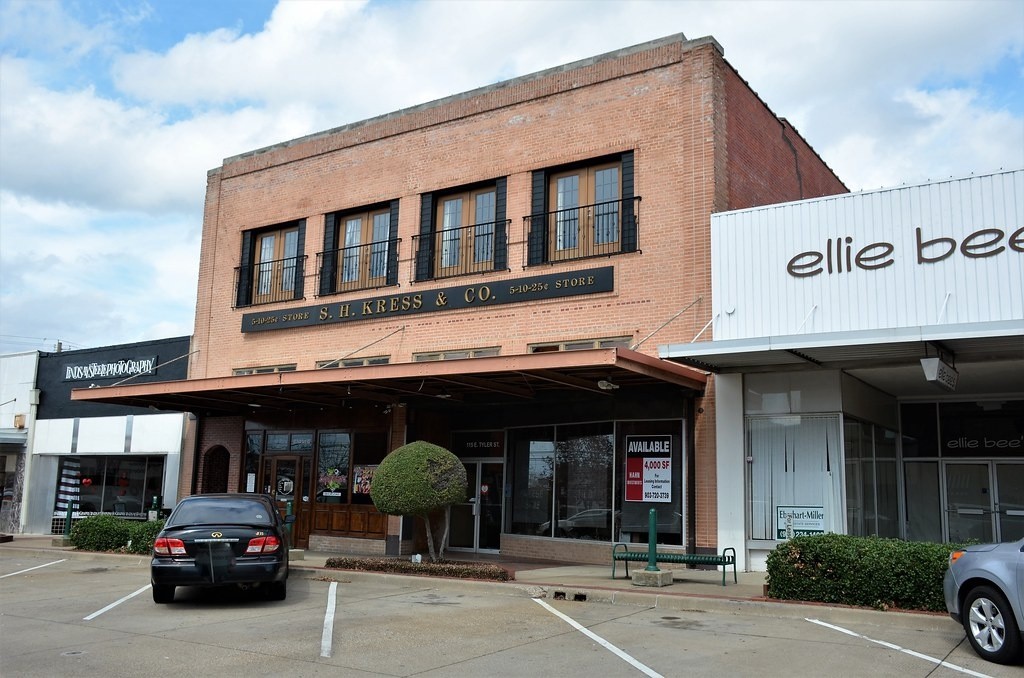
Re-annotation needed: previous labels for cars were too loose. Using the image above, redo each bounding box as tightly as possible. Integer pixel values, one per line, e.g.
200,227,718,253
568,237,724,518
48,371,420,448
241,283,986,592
150,494,295,603
944,534,1024,665
539,509,607,537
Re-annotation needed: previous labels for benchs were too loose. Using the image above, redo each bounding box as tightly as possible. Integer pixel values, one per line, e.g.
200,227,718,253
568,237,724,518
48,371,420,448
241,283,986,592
612,543,738,586
